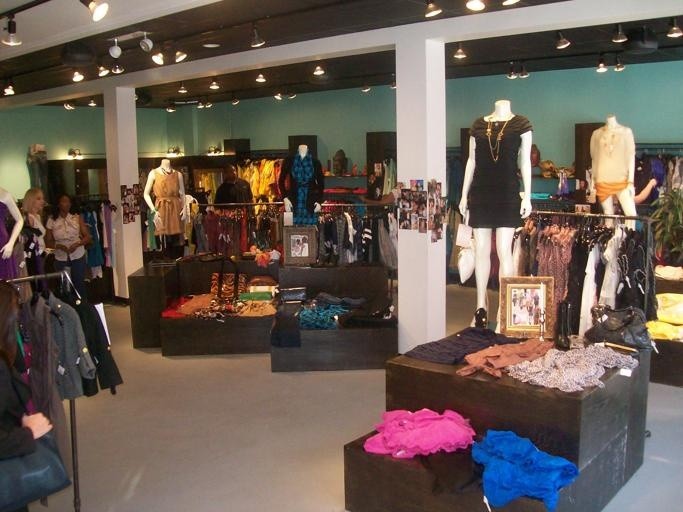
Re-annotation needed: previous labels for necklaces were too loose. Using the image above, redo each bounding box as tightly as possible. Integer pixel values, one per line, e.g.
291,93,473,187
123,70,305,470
485,121,508,162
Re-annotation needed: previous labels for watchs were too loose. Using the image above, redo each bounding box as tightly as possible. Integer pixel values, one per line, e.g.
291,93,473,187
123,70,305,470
77,241,82,245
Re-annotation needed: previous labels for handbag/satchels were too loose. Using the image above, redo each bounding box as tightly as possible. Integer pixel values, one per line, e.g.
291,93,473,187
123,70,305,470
585,304,654,349
0,355,72,512
455,210,475,284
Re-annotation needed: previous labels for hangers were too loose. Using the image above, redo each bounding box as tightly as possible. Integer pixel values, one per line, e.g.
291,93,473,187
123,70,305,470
78,192,109,201
59,273,69,292
199,202,284,213
515,211,651,441
530,211,648,230
8,275,23,290
42,273,50,300
188,201,396,274
0,266,82,512
322,204,390,214
637,148,683,154
20,275,64,327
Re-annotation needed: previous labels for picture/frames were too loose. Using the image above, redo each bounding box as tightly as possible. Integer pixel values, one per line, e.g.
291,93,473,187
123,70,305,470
285,226,316,264
500,275,554,339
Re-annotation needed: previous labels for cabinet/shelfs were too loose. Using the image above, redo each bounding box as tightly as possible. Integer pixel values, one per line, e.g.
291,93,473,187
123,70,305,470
127,257,176,349
534,173,576,212
271,264,397,373
652,264,683,386
163,260,279,357
343,327,651,512
323,174,368,188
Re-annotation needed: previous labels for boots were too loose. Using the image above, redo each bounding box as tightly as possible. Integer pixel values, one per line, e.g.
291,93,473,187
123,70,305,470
556,301,570,349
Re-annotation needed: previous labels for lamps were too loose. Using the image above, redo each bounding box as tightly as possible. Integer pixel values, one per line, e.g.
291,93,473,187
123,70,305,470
314,63,324,74
519,65,529,78
232,95,239,105
362,81,370,92
152,53,164,65
667,18,683,38
614,58,625,71
109,45,122,59
251,27,266,48
88,96,97,107
287,90,296,99
140,38,153,52
454,43,466,60
596,59,608,73
166,100,176,113
64,100,75,110
556,31,570,49
256,71,266,82
390,81,396,89
96,63,110,77
274,90,281,100
178,81,187,93
209,78,219,89
507,66,517,79
197,101,204,108
205,99,212,108
4,84,14,96
467,0,485,11
111,61,124,73
71,66,84,82
1,22,22,46
423,1,442,18
80,0,108,21
174,49,187,62
613,24,627,43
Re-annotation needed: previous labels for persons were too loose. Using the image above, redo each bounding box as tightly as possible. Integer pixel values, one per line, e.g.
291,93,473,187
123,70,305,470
634,179,656,204
44,193,93,297
19,188,47,289
0,280,53,512
359,188,397,205
213,162,254,211
